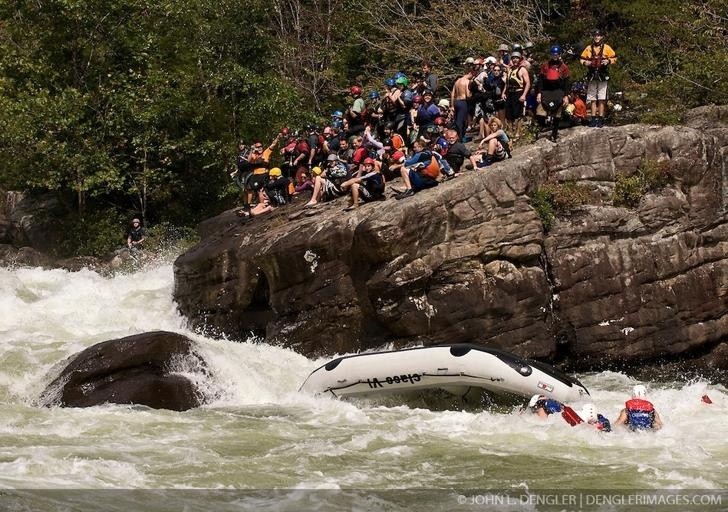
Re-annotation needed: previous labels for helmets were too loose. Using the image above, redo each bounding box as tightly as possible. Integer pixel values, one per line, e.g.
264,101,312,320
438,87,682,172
631,385,647,400
528,394,544,415
460,44,523,69
237,72,450,179
592,30,603,36
551,45,560,55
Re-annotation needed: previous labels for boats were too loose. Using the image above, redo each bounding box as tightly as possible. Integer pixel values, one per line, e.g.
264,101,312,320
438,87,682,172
297,343,597,416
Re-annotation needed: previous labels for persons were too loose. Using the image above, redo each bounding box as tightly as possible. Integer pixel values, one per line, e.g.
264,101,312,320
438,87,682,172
528,392,585,426
579,403,613,433
127,217,144,273
611,385,663,434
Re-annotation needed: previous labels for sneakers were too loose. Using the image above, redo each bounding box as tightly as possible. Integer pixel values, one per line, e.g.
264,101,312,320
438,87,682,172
390,186,405,193
589,123,596,127
395,188,414,200
597,122,603,127
